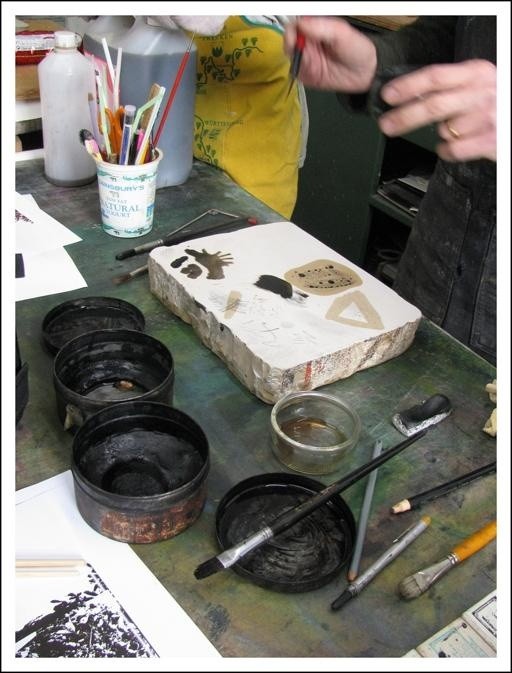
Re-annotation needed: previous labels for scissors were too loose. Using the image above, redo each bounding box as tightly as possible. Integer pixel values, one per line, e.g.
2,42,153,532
98,107,126,164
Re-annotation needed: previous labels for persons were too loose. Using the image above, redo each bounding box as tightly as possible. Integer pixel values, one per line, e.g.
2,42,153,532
277,16,496,363
17,15,313,228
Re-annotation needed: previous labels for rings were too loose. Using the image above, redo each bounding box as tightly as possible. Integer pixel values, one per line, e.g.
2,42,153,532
445,119,461,140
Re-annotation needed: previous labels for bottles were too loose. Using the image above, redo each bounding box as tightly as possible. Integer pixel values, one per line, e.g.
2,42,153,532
35,29,96,188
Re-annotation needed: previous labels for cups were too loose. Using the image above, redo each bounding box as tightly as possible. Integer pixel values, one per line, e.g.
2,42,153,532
270,390,361,475
100,143,164,237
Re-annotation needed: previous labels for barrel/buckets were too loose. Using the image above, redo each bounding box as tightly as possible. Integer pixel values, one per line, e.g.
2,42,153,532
81,16,199,190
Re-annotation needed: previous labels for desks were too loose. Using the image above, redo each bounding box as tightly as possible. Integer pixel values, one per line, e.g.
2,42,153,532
14,159,498,657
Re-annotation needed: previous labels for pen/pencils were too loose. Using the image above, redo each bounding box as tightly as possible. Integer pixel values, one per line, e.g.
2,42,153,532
348,440,382,582
391,463,497,514
331,516,432,611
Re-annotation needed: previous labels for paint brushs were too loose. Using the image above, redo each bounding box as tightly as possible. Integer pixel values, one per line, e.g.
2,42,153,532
398,519,497,600
112,264,149,286
285,30,306,99
193,430,427,580
79,31,195,164
116,214,252,260
